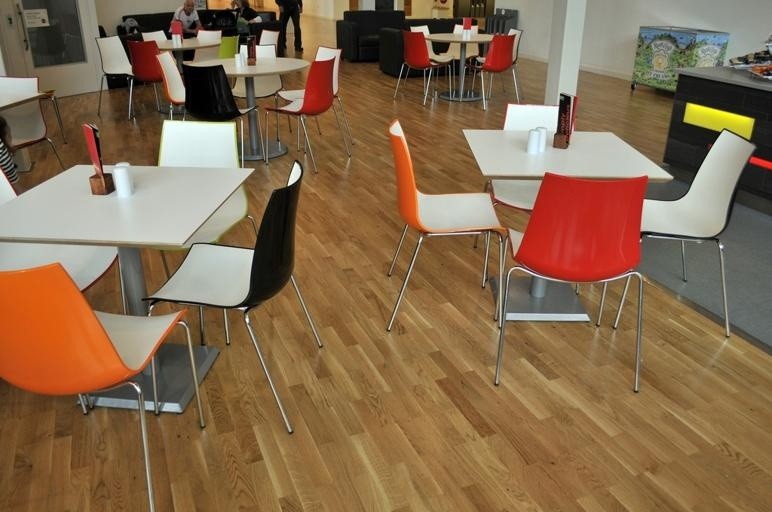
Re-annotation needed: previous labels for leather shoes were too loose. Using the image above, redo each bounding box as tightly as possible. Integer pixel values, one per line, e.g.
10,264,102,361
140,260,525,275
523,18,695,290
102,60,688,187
295,47,303,52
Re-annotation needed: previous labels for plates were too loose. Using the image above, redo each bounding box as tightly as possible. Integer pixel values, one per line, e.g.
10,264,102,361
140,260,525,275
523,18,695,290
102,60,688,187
748,68,772,81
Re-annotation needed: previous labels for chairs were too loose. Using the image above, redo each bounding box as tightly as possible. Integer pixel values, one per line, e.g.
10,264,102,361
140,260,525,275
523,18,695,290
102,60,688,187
232,44,292,140
122,120,257,346
278,46,354,153
182,64,266,168
0,77,68,188
0,169,129,316
387,118,506,332
143,162,323,433
95,29,239,121
1,264,206,511
595,128,758,338
393,26,523,110
264,57,351,173
494,173,648,394
474,103,575,280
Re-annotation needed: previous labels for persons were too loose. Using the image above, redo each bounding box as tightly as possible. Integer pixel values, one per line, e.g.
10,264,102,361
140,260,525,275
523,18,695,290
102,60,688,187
166,1,202,58
274,0,304,58
231,0,262,44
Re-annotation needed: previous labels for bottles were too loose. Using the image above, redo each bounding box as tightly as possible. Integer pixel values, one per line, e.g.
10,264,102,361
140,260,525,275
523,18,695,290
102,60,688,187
235,54,242,69
537,127,546,150
113,166,129,199
466,30,470,40
171,34,176,44
176,34,181,44
463,30,467,40
115,161,134,190
526,130,538,154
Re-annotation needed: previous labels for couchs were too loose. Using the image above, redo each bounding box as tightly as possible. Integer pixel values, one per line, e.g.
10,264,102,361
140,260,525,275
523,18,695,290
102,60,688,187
379,27,485,78
336,10,477,63
118,10,240,41
250,12,286,56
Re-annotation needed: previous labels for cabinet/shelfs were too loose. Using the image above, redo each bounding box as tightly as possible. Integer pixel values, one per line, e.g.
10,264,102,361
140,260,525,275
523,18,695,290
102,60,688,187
631,26,730,93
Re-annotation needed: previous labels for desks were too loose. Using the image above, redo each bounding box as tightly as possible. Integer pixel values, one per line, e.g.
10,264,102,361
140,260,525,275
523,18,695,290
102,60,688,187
0,165,255,414
0,93,46,112
183,58,310,162
662,62,772,199
463,129,674,322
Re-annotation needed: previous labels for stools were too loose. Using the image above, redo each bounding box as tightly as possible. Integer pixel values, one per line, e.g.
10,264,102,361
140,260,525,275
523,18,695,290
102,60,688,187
42,89,68,145
